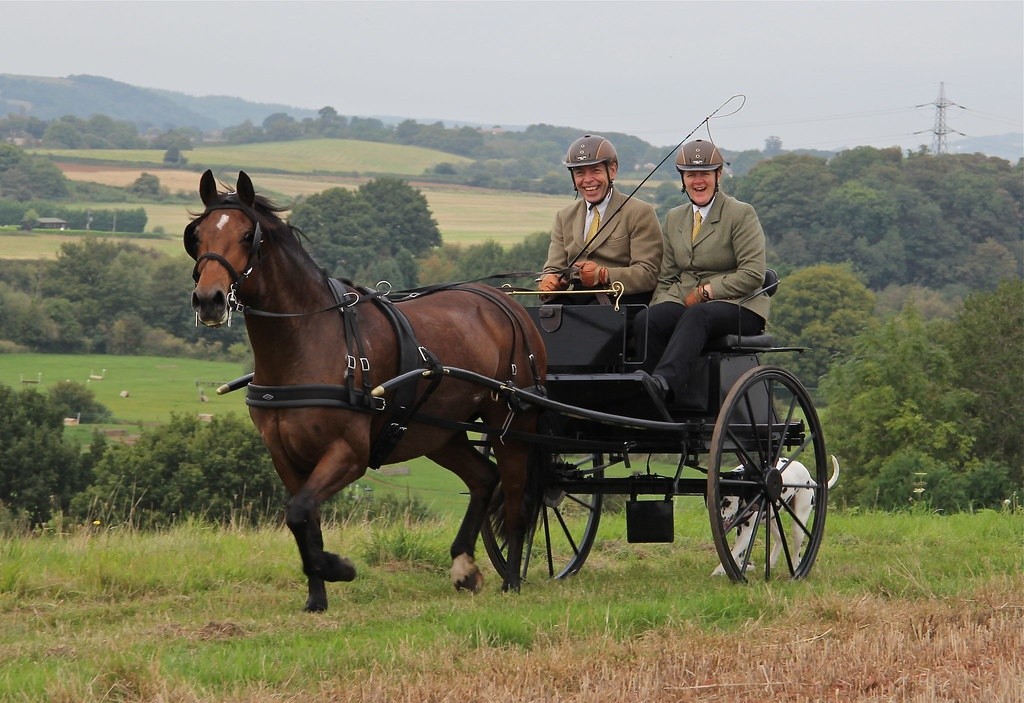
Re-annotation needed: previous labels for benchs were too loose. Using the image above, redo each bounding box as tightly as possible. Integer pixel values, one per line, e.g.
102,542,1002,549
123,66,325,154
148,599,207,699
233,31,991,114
717,268,780,351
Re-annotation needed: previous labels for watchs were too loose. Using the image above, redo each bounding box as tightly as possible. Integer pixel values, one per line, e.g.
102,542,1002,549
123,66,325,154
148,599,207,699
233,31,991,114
703,285,712,301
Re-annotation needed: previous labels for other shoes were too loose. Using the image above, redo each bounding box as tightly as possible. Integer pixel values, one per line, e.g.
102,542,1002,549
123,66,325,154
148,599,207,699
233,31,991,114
636,369,670,411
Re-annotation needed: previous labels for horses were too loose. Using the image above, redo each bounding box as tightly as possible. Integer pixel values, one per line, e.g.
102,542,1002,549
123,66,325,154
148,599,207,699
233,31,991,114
182,168,549,614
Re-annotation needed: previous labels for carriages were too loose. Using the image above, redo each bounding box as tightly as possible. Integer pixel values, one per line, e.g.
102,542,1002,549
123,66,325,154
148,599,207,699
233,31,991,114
183,169,828,615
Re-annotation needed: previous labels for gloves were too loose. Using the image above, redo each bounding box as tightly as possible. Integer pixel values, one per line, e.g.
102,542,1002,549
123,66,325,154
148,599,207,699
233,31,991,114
574,261,608,288
538,274,567,301
685,286,708,306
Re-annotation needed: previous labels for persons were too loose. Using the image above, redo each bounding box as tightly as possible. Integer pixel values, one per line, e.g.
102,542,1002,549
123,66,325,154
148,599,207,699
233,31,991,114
535,133,663,340
630,139,770,406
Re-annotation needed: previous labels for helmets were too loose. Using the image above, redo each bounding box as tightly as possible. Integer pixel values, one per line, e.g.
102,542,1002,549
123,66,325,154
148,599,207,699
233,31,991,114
676,140,724,171
565,135,617,166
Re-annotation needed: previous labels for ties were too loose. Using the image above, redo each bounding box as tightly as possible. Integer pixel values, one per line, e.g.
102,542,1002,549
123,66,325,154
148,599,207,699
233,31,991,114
692,211,701,242
586,207,599,245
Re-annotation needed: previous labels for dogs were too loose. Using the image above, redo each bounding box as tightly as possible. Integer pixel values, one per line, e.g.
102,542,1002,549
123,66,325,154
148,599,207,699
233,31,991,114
703,455,840,576
